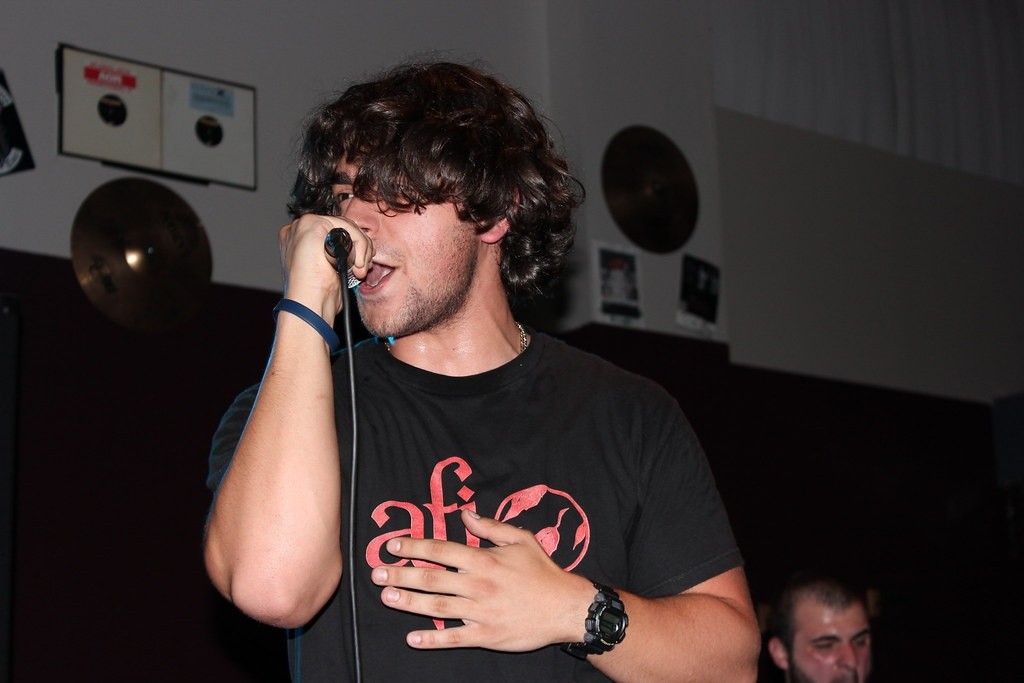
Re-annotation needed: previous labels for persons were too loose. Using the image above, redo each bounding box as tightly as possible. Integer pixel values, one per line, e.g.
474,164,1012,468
765,566,876,683
202,64,761,683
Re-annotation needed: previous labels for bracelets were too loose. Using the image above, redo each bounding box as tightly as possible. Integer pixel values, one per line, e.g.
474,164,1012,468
272,299,339,349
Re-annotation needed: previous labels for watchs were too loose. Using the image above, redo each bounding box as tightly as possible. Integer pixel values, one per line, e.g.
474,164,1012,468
559,577,628,660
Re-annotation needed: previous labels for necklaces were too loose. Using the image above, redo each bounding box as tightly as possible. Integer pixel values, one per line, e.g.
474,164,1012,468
385,319,527,357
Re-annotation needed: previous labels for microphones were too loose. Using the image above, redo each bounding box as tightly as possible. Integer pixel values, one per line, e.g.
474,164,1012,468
324,226,363,289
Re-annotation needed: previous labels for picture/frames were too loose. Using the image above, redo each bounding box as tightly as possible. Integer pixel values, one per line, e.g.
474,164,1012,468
588,239,647,329
681,255,721,324
55,42,258,192
